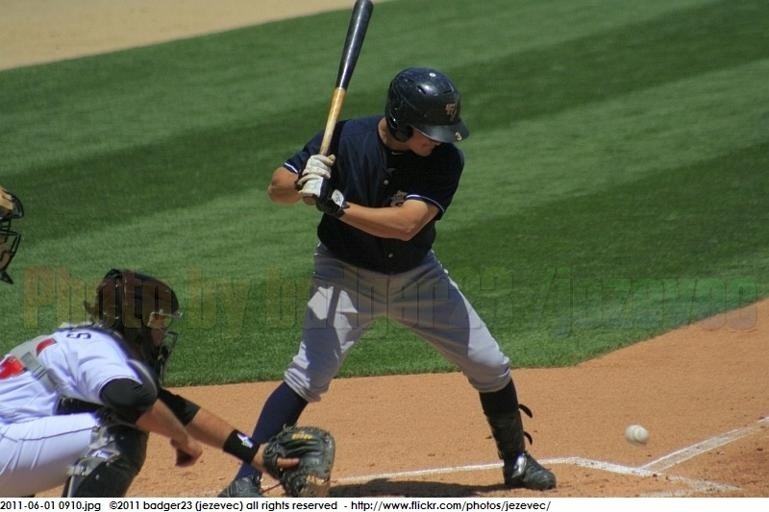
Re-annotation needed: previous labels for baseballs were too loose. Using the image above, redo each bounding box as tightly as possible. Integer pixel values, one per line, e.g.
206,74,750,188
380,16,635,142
625,424,649,445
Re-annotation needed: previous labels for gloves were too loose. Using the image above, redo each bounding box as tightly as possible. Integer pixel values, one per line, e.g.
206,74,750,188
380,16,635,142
294,153,337,194
295,174,350,219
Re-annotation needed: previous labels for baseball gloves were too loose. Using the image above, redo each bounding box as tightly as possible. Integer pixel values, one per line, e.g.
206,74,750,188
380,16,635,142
262,425,335,498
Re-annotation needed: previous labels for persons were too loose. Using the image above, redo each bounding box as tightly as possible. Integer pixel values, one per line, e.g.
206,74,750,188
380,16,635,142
0,267,335,498
220,66,557,498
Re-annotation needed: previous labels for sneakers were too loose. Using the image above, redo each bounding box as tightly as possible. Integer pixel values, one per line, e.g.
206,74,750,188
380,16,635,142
502,451,557,491
218,475,262,497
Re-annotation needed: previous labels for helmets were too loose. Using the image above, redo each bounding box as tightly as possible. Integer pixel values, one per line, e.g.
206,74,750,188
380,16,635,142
383,67,472,144
88,268,184,368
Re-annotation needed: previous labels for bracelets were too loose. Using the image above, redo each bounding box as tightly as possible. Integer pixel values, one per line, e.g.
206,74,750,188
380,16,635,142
223,429,260,465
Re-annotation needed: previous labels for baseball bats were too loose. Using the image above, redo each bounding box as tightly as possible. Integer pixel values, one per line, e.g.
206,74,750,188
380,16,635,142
303,0,373,204
